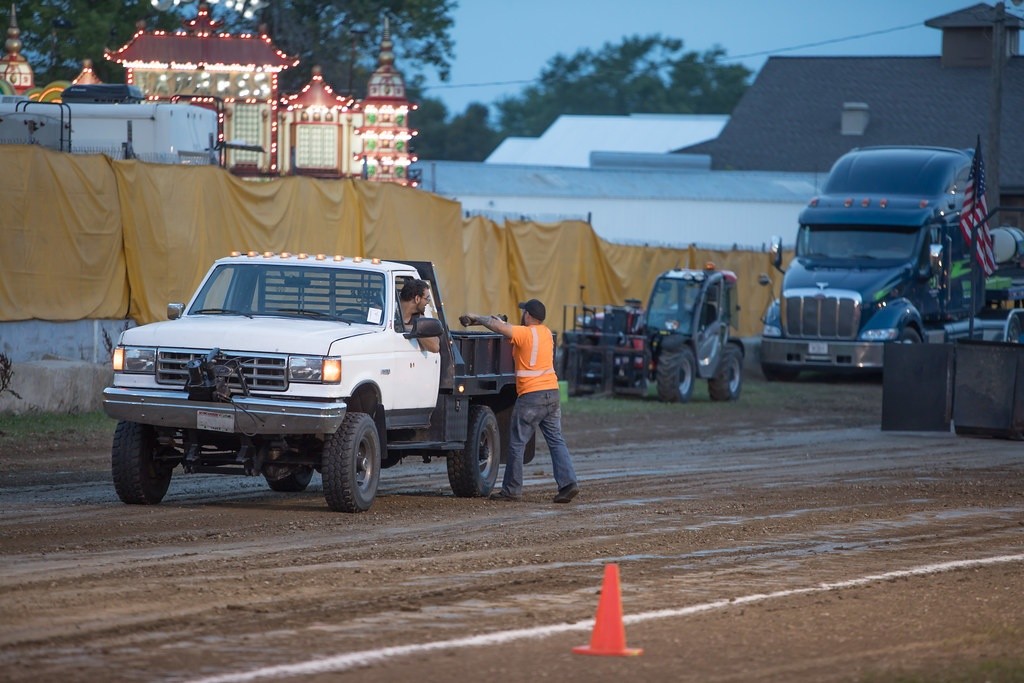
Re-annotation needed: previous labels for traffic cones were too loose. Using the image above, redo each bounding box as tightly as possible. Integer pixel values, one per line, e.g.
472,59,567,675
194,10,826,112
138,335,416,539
572,563,643,656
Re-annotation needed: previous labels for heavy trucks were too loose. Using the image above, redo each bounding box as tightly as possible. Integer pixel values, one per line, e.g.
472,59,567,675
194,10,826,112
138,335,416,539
758,144,1024,380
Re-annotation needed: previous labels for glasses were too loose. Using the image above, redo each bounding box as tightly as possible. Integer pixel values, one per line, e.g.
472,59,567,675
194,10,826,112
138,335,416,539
418,294,431,302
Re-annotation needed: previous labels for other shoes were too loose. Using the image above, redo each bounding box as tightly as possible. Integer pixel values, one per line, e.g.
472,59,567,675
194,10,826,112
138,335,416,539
553,483,579,504
490,492,522,502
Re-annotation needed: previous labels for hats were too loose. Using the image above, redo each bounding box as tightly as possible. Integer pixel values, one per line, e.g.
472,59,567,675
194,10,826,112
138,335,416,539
519,299,546,321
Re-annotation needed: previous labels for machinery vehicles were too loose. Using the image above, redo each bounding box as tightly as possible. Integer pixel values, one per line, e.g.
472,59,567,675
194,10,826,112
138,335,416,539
552,264,744,401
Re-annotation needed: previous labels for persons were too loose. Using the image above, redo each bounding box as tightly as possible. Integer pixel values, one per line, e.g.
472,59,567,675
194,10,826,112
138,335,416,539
464,299,579,503
376,280,440,354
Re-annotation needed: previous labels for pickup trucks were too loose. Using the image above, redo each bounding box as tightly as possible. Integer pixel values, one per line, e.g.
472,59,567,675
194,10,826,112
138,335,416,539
102,251,536,513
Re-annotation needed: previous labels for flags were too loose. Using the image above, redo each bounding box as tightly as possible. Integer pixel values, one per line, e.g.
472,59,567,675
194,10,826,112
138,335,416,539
960,143,997,276
